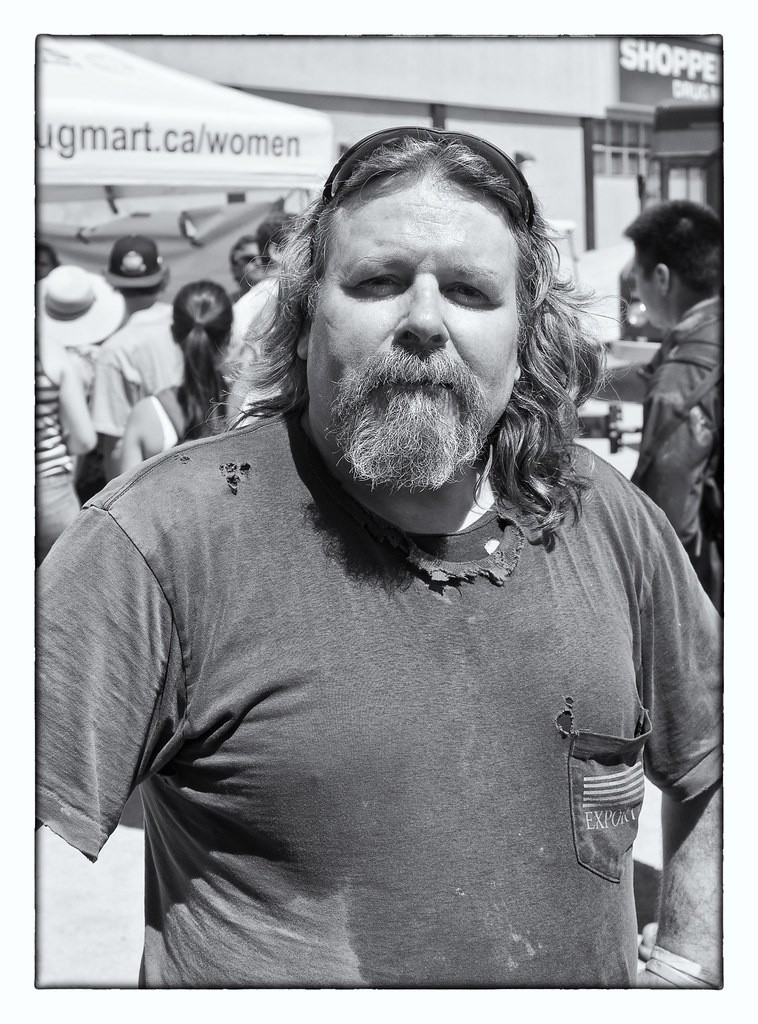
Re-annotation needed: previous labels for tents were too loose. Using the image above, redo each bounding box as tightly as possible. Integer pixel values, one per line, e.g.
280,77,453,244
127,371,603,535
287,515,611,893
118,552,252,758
38,35,337,217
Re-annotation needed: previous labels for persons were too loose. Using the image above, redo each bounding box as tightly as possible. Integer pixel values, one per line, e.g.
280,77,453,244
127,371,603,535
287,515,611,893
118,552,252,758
36,128,725,991
626,201,727,963
36,210,299,565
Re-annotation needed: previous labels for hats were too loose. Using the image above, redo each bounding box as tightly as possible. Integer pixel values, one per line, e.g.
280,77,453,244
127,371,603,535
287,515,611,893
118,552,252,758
106,235,163,287
34,266,124,346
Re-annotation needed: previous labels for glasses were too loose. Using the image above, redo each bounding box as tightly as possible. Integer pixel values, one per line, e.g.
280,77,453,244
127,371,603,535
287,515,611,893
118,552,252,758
235,255,262,263
308,126,535,266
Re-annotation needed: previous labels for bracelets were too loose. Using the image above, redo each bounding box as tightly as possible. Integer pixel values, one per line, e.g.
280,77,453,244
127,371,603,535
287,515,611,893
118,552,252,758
645,945,723,989
